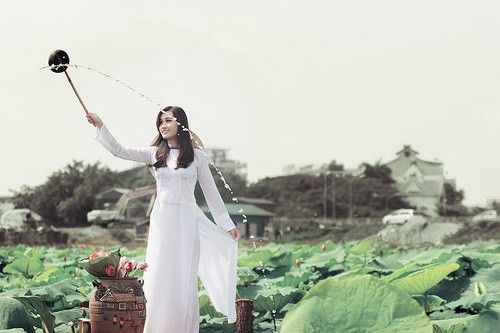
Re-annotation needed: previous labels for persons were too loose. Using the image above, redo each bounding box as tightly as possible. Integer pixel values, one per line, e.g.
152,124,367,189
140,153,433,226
86,105,240,333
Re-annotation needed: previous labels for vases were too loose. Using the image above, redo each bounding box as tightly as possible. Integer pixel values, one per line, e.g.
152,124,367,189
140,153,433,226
89,278,147,333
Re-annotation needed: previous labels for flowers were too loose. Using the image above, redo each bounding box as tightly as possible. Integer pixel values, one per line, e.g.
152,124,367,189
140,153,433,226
81,249,148,279
